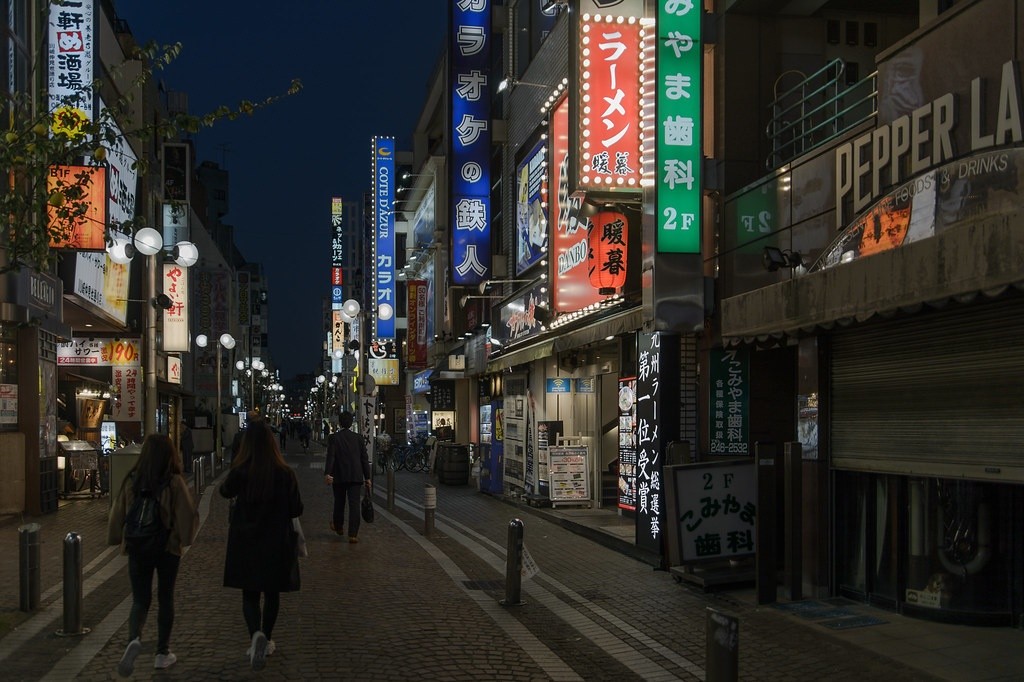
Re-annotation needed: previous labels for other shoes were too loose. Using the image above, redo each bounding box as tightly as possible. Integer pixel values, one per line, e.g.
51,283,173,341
266,639,276,655
247,631,268,672
349,536,360,544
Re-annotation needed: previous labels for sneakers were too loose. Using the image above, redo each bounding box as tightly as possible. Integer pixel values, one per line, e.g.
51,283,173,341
117,638,143,678
153,651,177,668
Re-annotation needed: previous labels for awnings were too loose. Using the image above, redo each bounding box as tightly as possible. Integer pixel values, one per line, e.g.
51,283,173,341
719,205,1024,347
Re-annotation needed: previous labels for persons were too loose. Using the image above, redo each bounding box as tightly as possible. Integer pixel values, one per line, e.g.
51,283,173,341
300,417,312,450
296,419,303,435
324,412,372,543
289,419,295,441
287,420,291,436
106,433,200,679
276,418,289,450
219,420,304,672
322,419,330,445
231,410,259,470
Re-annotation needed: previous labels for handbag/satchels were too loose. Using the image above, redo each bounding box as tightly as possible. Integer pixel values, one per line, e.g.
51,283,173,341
361,484,376,523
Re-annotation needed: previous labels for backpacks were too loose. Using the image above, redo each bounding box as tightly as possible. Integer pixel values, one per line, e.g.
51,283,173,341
123,487,173,555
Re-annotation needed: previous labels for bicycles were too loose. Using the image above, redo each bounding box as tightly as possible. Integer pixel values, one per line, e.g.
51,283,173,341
378,433,432,473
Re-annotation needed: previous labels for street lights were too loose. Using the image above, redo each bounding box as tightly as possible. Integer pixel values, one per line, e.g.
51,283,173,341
196,334,235,465
107,229,199,436
318,375,338,418
342,299,393,435
236,360,265,410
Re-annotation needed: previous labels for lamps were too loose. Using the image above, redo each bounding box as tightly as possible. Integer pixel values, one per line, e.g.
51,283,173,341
533,305,555,330
392,200,423,205
128,317,137,329
464,329,475,336
401,170,435,180
458,294,505,309
481,320,491,328
434,334,444,342
540,0,571,14
488,336,509,348
396,185,429,193
478,279,534,294
457,335,470,340
403,261,423,269
763,246,804,271
442,330,452,338
409,251,429,260
386,209,415,216
494,74,557,95
399,268,417,276
